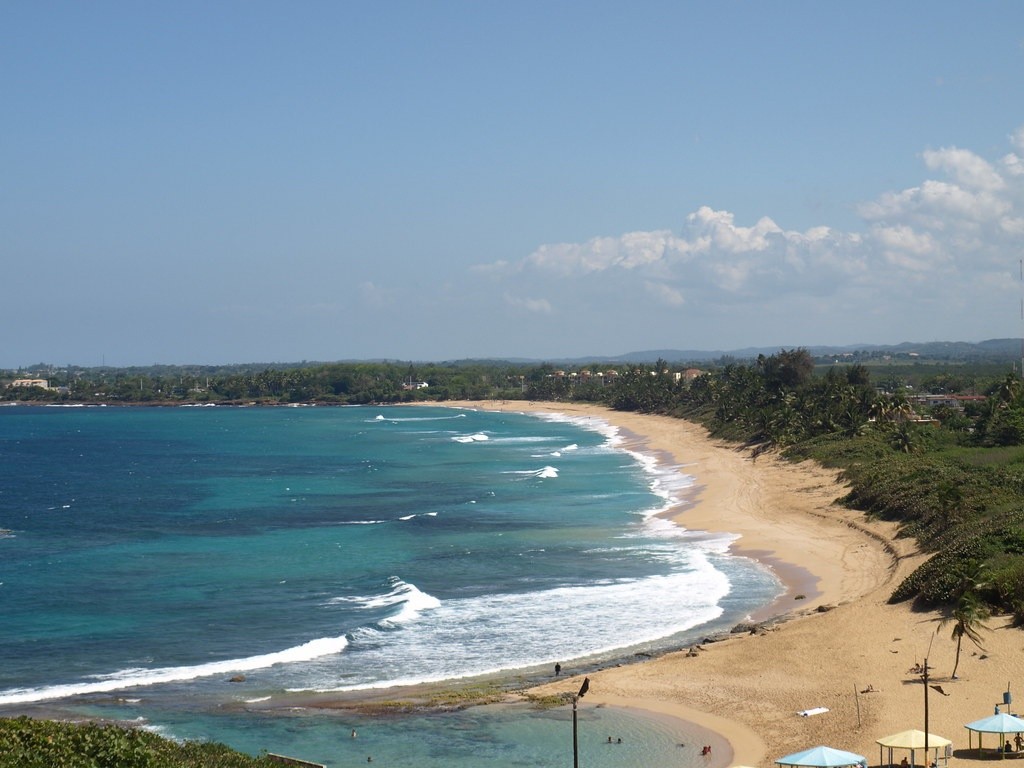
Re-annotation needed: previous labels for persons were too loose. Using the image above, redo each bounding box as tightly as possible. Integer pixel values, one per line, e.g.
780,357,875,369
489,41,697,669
1014,733,1023,751
901,757,908,768
555,663,560,677
352,729,356,736
995,704,1000,715
914,663,920,674
703,746,711,755
608,737,613,742
1005,740,1012,751
367,756,372,762
617,738,622,744
